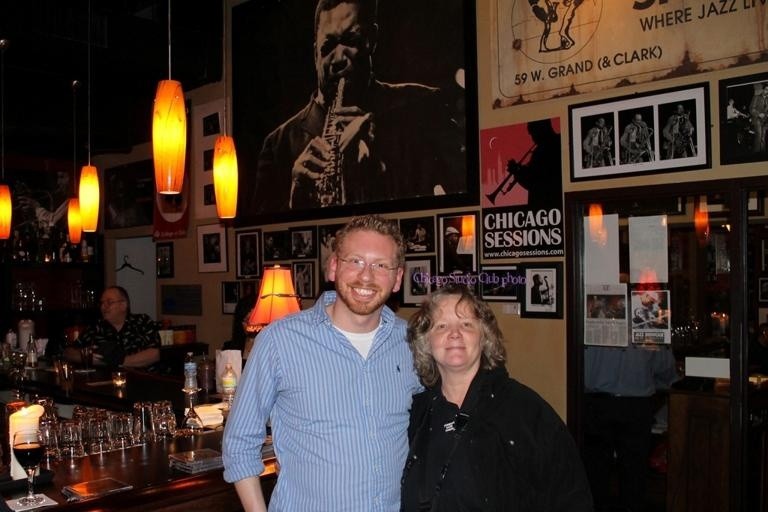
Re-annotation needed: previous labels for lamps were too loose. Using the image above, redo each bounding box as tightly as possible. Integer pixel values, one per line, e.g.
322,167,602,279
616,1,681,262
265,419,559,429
66,78,82,242
155,0,189,196
242,264,300,340
0,39,11,242
212,1,241,218
78,1,101,230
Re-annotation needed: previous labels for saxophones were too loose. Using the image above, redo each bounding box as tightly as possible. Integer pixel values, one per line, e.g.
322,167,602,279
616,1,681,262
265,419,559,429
592,124,613,160
628,127,654,164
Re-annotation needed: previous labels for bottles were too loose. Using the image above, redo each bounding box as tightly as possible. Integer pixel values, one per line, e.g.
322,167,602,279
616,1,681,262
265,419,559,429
7,329,17,349
221,355,237,418
198,353,215,391
27,333,39,368
184,353,197,393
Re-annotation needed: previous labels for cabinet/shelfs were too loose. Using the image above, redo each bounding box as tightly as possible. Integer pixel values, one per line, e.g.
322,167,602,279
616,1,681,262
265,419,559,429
4,214,108,340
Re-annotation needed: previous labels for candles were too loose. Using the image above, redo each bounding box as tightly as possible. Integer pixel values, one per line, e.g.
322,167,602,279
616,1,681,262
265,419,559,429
10,406,45,480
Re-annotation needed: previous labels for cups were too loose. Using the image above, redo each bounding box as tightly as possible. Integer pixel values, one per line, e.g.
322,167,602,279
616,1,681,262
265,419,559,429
57,363,74,379
13,430,46,507
111,370,129,388
180,387,204,437
10,351,27,369
37,397,177,463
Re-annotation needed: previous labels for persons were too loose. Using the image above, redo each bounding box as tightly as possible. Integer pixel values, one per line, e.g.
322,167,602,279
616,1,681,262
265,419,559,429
620,112,655,165
61,284,163,370
294,265,309,297
400,281,596,512
584,347,678,512
659,102,698,161
265,236,278,262
528,273,554,307
726,98,747,121
251,1,454,210
408,222,429,244
582,117,615,170
222,213,429,511
748,83,768,154
444,224,468,276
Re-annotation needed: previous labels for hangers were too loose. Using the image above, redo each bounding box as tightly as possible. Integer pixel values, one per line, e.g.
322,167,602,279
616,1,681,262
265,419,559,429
114,256,145,276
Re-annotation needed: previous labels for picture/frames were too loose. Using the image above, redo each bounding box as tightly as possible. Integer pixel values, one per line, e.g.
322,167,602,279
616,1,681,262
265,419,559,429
480,262,518,301
161,283,202,318
293,262,316,301
156,242,175,280
397,216,437,253
263,228,292,260
292,225,319,258
234,229,262,279
196,224,229,273
228,0,481,228
488,0,768,110
435,211,482,276
476,118,564,264
318,223,345,292
261,230,292,264
242,282,258,299
223,281,240,314
567,80,711,184
100,159,154,239
194,96,228,219
520,260,565,320
403,255,437,309
719,71,766,165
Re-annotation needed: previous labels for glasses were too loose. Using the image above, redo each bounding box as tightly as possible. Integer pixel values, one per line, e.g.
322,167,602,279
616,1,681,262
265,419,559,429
338,257,404,276
99,299,128,306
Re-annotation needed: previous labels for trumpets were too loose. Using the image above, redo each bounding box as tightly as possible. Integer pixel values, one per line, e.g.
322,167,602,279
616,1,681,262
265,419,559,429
485,143,537,206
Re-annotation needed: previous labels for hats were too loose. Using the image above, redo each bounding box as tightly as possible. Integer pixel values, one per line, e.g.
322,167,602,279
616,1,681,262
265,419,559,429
446,227,460,237
